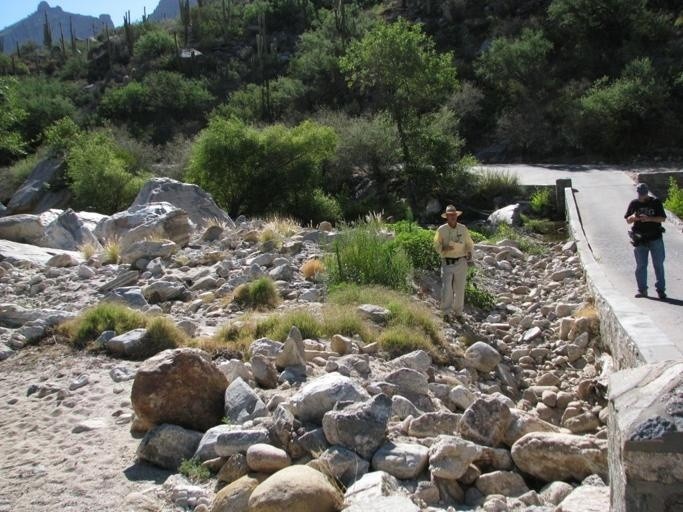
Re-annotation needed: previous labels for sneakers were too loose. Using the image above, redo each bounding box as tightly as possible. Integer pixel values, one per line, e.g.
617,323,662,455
633,289,648,298
455,314,465,324
442,314,451,323
657,290,667,300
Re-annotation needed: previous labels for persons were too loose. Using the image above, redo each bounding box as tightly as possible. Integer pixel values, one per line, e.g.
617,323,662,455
434,205,475,325
624,183,668,300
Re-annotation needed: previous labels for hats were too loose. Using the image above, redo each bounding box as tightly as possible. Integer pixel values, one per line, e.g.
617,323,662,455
440,203,464,219
637,183,649,194
